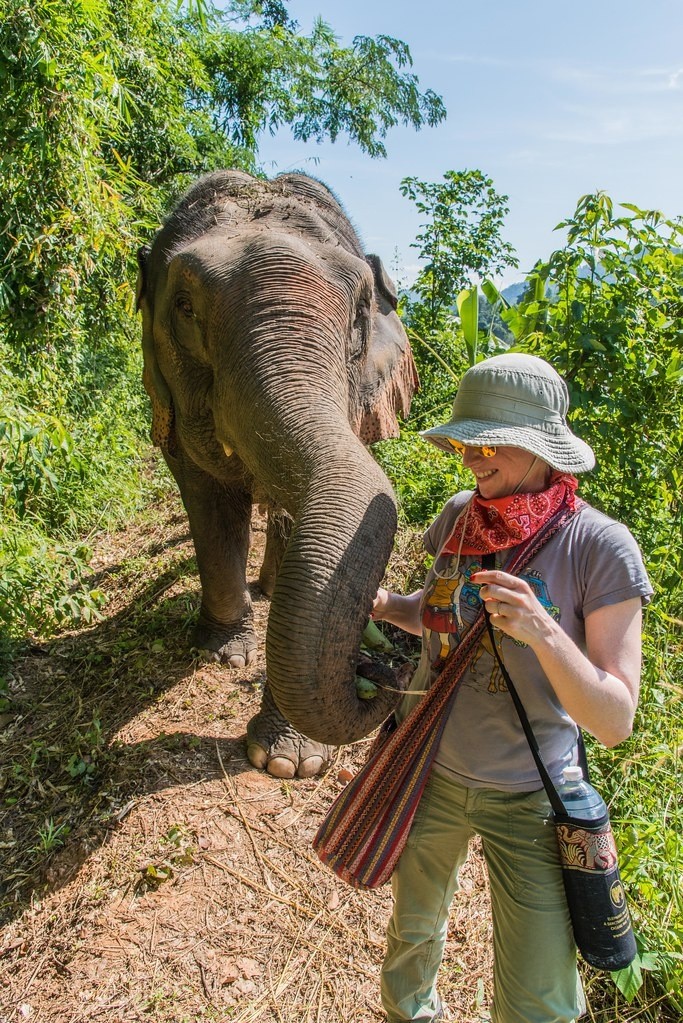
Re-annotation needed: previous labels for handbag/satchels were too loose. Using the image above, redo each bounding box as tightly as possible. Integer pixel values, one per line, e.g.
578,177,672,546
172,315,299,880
554,814,637,972
310,708,450,891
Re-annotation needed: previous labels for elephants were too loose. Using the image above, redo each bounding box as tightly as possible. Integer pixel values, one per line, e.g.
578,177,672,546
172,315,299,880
133,171,414,779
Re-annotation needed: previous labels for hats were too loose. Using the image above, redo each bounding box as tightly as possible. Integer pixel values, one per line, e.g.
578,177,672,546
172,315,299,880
417,351,596,474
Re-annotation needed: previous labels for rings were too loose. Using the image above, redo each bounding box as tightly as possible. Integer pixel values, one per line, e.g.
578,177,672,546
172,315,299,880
497,602,502,616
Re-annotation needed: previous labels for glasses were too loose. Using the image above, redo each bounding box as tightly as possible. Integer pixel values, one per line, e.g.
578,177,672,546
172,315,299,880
442,437,498,457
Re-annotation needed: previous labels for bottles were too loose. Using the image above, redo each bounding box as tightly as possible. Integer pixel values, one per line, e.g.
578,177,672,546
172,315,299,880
558,766,605,820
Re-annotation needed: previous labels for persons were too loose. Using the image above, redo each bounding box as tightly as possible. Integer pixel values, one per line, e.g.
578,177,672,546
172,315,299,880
369,354,654,1023
